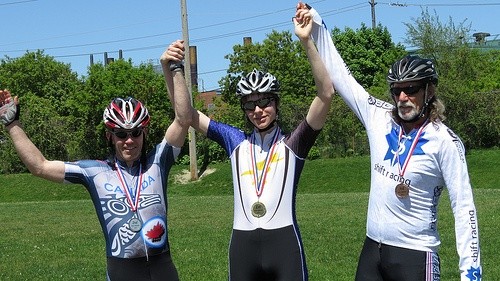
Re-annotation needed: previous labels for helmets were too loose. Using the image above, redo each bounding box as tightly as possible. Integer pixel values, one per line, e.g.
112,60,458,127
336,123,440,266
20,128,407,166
235,67,278,97
103,97,151,131
387,55,439,85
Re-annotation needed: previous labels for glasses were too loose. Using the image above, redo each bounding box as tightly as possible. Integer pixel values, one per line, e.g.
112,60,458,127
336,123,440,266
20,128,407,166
243,97,276,112
390,81,432,97
108,129,141,140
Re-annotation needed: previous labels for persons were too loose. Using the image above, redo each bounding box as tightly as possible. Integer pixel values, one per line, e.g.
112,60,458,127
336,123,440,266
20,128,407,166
296,1,482,281
160,9,335,281
0,39,193,281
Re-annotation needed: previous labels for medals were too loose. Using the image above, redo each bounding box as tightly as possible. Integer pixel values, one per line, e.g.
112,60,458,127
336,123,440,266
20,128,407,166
128,217,142,231
395,183,409,199
252,202,267,218
